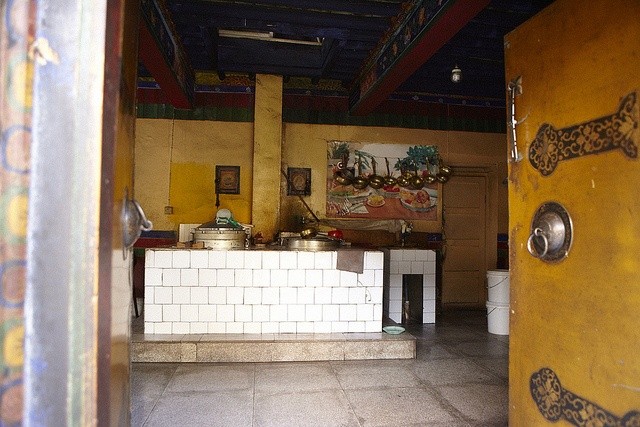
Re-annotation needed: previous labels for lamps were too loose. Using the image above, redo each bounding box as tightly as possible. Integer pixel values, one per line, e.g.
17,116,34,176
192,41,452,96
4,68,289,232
449,65,463,85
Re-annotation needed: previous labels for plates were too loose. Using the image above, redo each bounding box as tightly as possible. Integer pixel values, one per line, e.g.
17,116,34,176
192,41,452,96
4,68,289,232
383,325,405,335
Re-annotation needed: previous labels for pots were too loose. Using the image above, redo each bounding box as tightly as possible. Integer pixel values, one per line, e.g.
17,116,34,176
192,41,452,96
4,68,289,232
121,186,153,261
352,157,368,190
299,227,344,239
336,228,390,244
335,155,353,185
425,156,436,185
383,157,397,186
368,156,384,189
397,158,410,186
437,157,448,184
410,159,424,190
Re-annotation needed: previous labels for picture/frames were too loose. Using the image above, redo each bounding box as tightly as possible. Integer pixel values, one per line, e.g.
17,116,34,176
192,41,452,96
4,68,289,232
215,164,240,195
324,139,443,235
287,166,312,196
